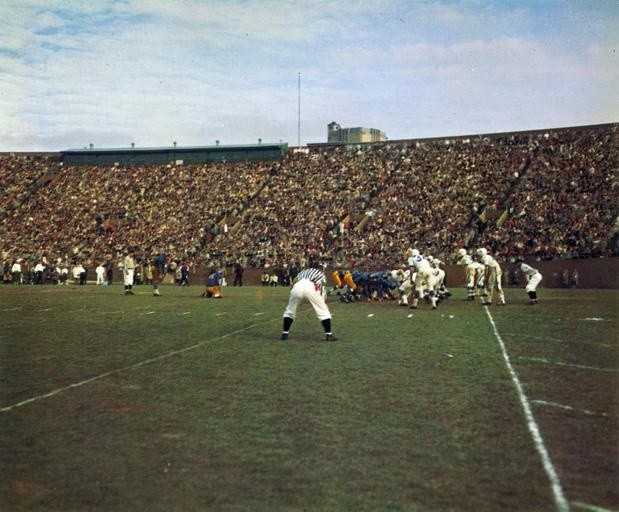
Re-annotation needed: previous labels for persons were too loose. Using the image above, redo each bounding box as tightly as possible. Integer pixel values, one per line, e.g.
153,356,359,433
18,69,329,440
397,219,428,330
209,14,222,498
281,263,338,341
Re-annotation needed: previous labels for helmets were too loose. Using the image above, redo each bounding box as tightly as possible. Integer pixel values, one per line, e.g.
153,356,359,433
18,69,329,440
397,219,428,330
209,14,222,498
457,248,467,257
475,247,487,257
411,248,420,257
459,257,471,267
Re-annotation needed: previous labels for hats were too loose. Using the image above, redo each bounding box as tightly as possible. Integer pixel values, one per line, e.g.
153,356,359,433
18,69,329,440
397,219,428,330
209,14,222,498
510,258,521,265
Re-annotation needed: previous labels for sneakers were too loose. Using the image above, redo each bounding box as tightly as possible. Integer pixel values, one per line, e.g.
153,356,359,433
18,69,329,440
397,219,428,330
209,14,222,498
466,295,507,307
279,331,290,341
325,332,339,342
124,289,135,295
524,299,539,306
153,289,161,296
326,291,452,310
